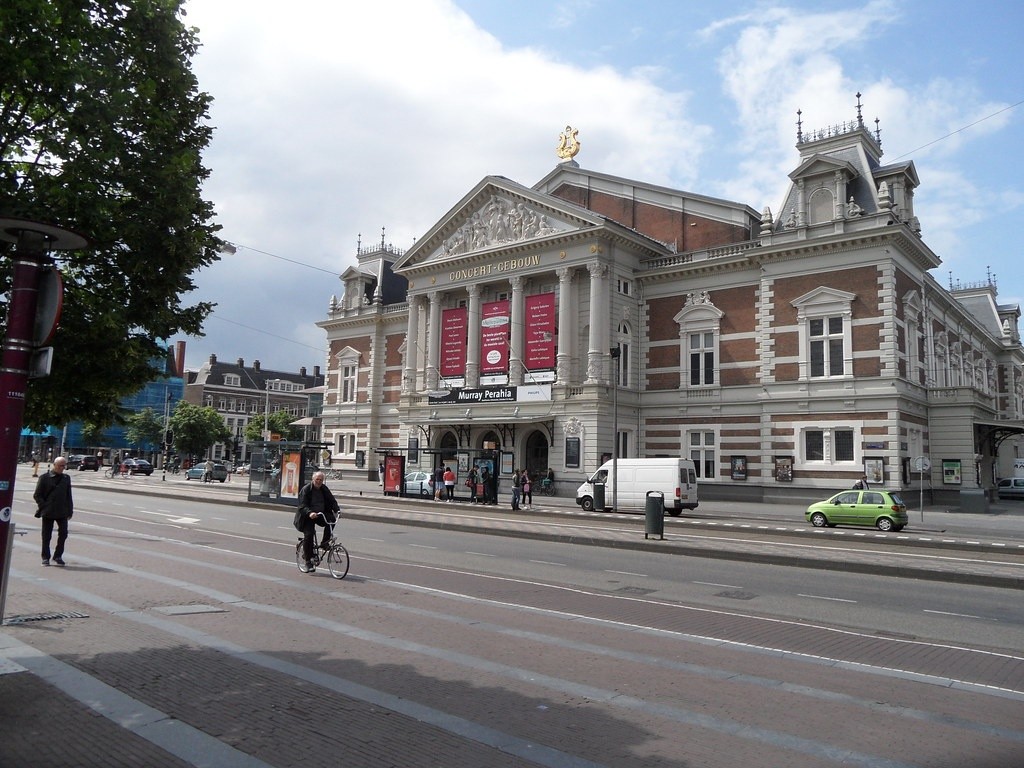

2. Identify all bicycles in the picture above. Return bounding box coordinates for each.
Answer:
[531,478,555,496]
[169,464,181,475]
[199,470,215,483]
[296,512,349,580]
[105,465,134,480]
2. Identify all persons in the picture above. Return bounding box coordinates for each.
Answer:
[547,468,554,486]
[204,458,214,481]
[520,468,533,510]
[468,465,479,504]
[378,463,385,486]
[443,467,455,503]
[511,469,521,510]
[113,453,120,475]
[481,467,492,505]
[293,471,340,572]
[442,194,553,257]
[853,475,869,490]
[434,463,445,501]
[33,457,73,567]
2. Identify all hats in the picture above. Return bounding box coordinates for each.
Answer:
[862,475,867,479]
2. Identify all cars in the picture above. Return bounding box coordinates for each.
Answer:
[185,462,227,483]
[997,478,1024,493]
[404,472,445,499]
[222,460,250,475]
[122,458,154,476]
[804,490,908,532]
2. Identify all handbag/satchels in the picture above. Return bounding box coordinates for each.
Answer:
[465,480,473,487]
[524,484,529,492]
[211,467,215,472]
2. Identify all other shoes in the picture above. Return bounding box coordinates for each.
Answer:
[305,562,315,571]
[42,559,49,566]
[320,543,332,551]
[53,556,65,565]
[513,507,521,510]
[450,499,453,502]
[434,497,440,501]
[446,500,450,502]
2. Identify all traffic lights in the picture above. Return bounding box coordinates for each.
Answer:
[166,430,173,445]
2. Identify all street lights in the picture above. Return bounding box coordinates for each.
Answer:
[264,379,280,441]
[208,395,214,406]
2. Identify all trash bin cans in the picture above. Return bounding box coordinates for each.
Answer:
[644,491,665,534]
[593,481,605,509]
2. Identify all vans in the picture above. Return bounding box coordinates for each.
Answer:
[67,455,98,472]
[576,458,699,516]
